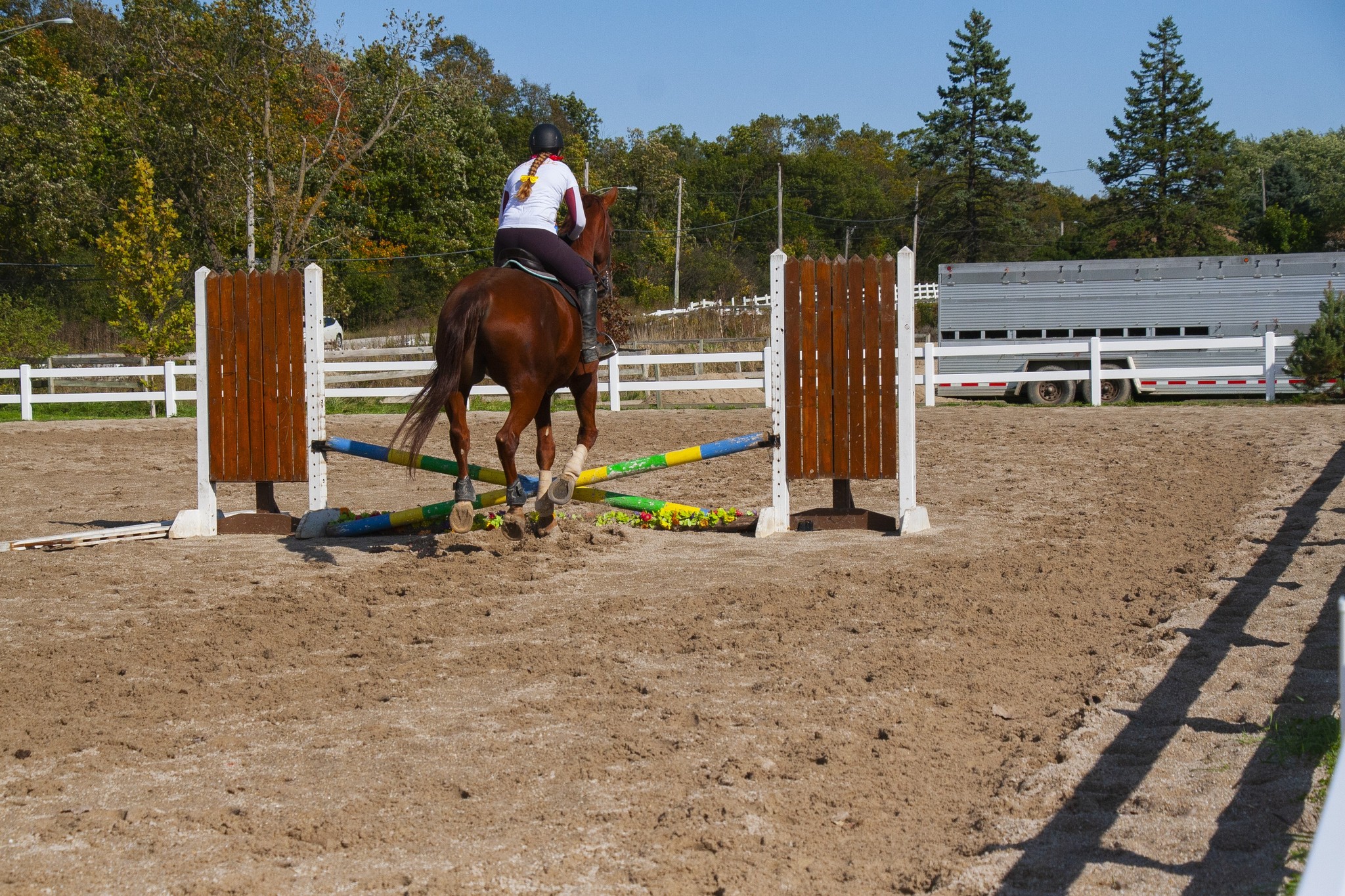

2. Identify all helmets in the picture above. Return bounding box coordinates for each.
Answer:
[528,124,564,151]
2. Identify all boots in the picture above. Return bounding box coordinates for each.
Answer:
[576,279,620,364]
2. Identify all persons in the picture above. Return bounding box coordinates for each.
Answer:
[493,123,619,363]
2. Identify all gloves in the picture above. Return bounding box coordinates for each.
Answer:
[558,229,580,247]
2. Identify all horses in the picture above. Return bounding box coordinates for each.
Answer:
[395,185,619,546]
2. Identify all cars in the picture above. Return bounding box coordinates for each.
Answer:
[290,315,344,351]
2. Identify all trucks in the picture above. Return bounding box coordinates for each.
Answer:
[936,250,1345,407]
